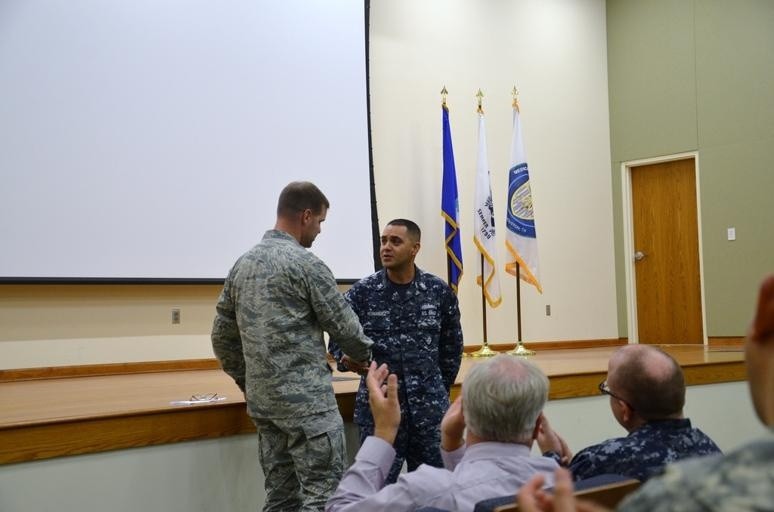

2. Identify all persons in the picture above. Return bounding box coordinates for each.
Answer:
[536,344,725,486]
[515,271,774,512]
[324,352,563,512]
[329,218,464,496]
[210,181,375,512]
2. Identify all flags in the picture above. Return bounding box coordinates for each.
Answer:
[442,101,464,296]
[474,105,503,308]
[504,88,543,295]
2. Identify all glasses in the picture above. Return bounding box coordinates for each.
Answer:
[598,379,624,401]
